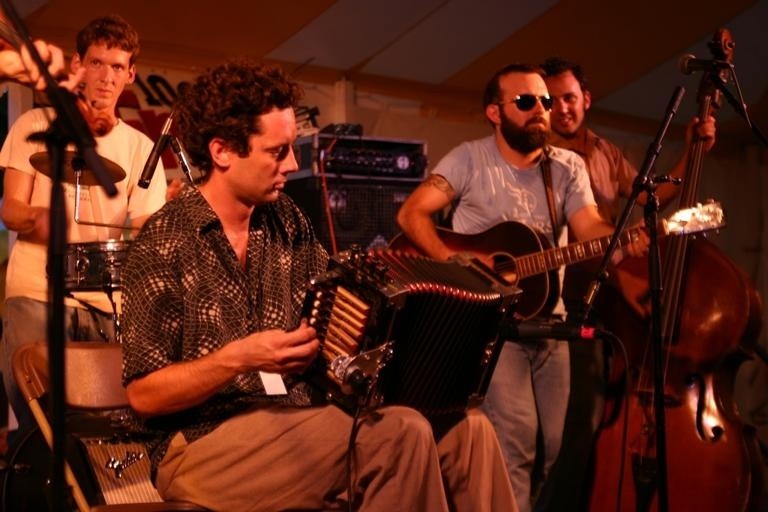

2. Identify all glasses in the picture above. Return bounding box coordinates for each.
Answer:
[498,94,554,111]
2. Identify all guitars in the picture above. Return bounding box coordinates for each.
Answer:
[387,198,726,323]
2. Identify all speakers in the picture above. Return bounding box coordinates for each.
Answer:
[285,178,444,256]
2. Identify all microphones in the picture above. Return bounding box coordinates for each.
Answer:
[505,319,606,342]
[679,53,734,74]
[135,110,175,188]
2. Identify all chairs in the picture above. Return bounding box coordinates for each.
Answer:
[10,339,210,512]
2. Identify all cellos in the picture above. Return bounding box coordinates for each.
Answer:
[563,27,764,510]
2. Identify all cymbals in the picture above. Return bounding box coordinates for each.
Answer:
[29,151,126,185]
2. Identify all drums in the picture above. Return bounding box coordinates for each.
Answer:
[57,239,131,292]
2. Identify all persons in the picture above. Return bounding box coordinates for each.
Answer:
[536,59,717,512]
[396,63,650,512]
[0,13,167,470]
[122,58,521,512]
